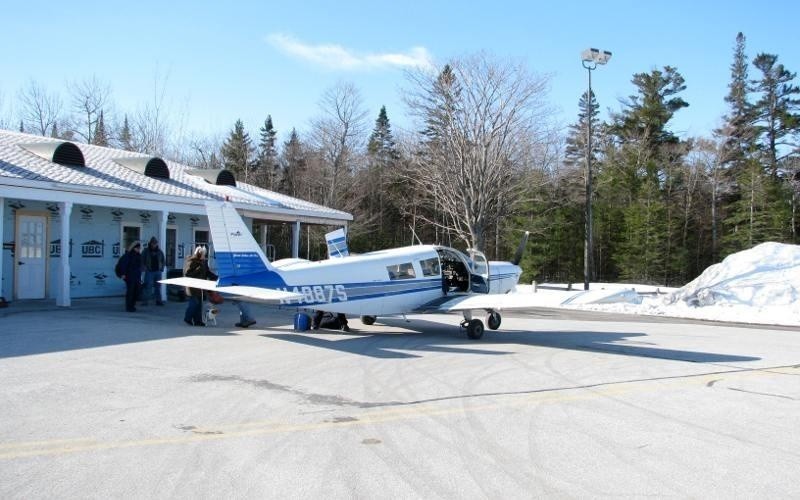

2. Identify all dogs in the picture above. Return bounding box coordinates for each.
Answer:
[204,301,219,327]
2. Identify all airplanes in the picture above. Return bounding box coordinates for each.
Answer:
[159,200,639,340]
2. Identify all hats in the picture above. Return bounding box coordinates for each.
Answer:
[150,236,158,244]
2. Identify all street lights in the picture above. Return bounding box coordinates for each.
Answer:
[582,48,611,289]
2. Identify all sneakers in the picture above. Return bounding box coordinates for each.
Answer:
[184,318,193,326]
[126,301,164,312]
[194,321,205,326]
[235,319,256,328]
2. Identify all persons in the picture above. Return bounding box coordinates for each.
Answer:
[182,246,217,326]
[142,237,166,306]
[115,241,141,312]
